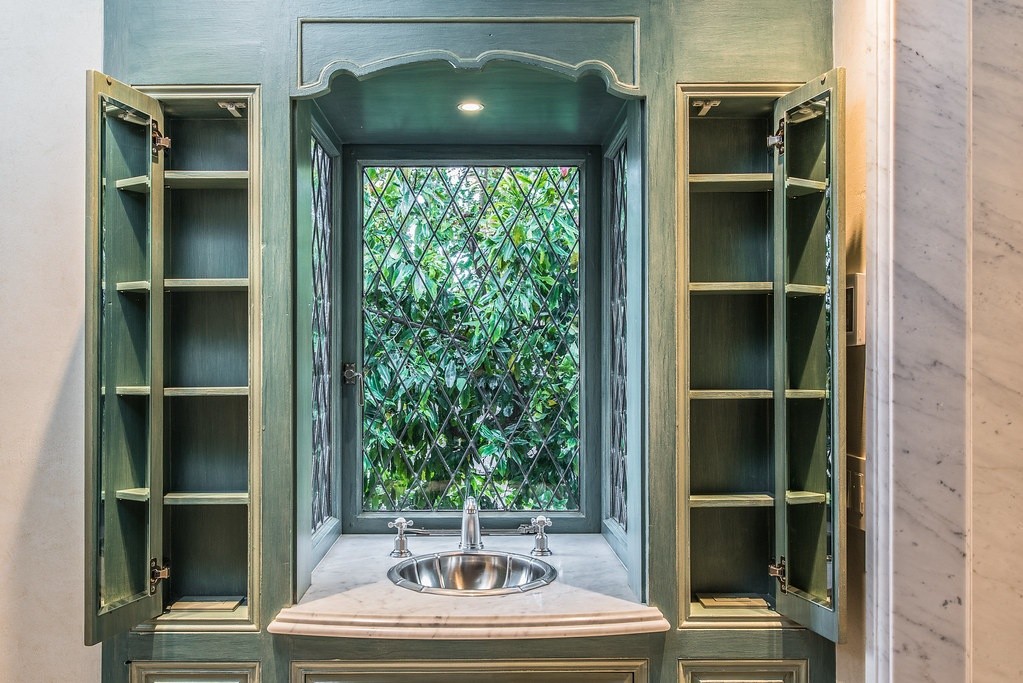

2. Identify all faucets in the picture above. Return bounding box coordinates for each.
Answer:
[458,496,485,550]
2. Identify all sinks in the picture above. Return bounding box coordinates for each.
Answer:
[386,549,558,597]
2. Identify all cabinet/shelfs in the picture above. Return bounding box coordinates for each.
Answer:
[82,69,263,647]
[672,66,850,643]
